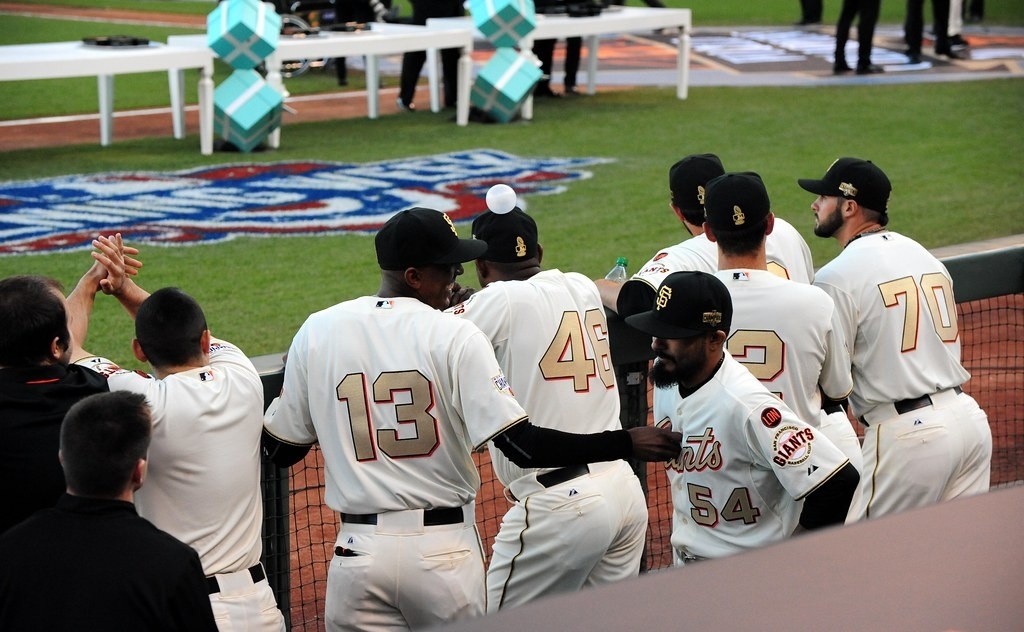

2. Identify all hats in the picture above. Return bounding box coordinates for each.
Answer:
[375,207,488,270]
[626,272,734,338]
[471,207,539,263]
[706,170,771,230]
[798,156,893,213]
[669,153,725,212]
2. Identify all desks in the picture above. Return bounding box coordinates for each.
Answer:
[0,5,693,156]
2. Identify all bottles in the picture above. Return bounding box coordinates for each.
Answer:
[605,257,628,282]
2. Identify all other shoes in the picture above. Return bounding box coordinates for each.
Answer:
[535,89,560,98]
[856,61,884,74]
[564,85,581,94]
[933,40,968,60]
[833,59,853,73]
[396,98,415,114]
[909,54,923,65]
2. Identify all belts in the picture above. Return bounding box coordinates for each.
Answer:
[509,464,589,501]
[340,507,463,526]
[204,562,263,592]
[822,402,843,415]
[857,384,963,428]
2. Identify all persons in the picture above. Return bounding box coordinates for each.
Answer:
[0,275,111,538]
[260,207,681,632]
[592,150,995,525]
[624,266,862,567]
[393,0,467,113]
[445,207,653,613]
[0,390,221,632]
[64,228,290,632]
[264,0,392,88]
[902,0,984,64]
[793,0,824,27]
[531,0,682,99]
[832,0,885,75]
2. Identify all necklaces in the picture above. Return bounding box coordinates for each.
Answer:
[677,353,725,397]
[843,226,885,248]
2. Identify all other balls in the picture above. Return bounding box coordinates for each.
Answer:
[486,183,517,214]
